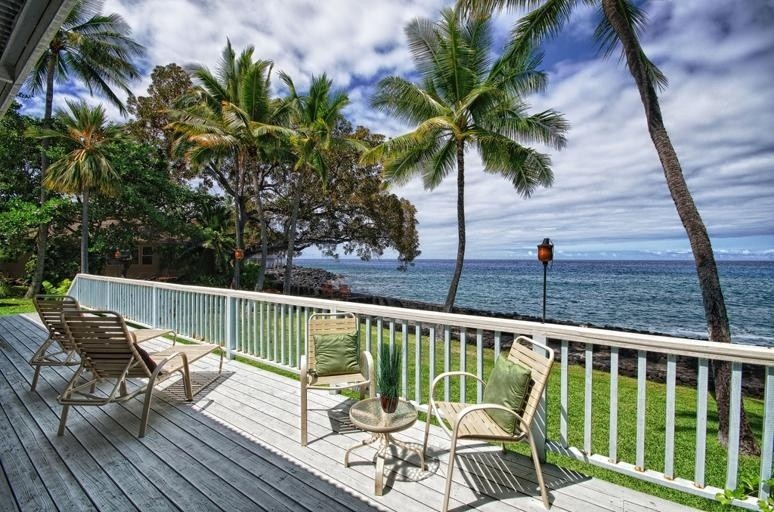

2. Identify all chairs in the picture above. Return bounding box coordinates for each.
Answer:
[424,335,555,512]
[300,312,375,446]
[55,310,223,438]
[28,294,177,393]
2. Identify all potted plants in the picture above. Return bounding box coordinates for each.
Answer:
[371,342,403,414]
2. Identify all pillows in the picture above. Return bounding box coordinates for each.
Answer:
[482,351,532,437]
[313,331,361,377]
